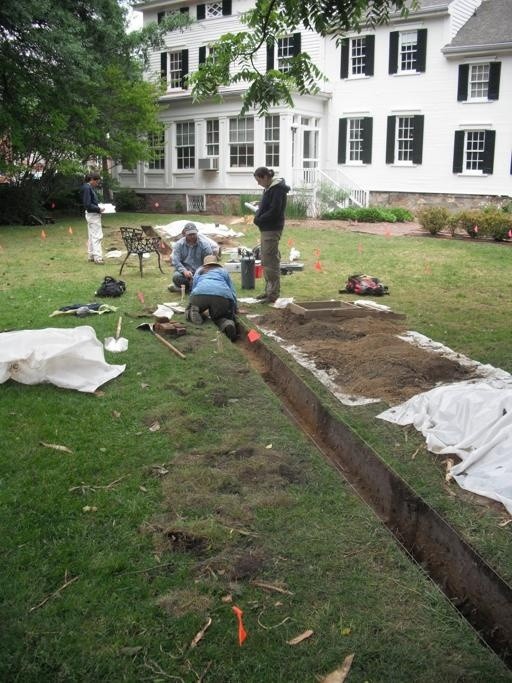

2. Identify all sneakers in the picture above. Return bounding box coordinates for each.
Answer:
[88,258,104,264]
[255,294,276,304]
[167,283,180,291]
[225,324,235,342]
[190,305,203,324]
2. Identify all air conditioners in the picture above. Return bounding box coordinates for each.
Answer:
[198,157,218,171]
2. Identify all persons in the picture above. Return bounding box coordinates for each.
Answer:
[253,166,287,304]
[170,223,238,341]
[81,174,105,264]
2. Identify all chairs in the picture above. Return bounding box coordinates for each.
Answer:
[119,226,164,278]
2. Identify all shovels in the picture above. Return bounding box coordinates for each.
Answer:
[136,323,187,361]
[104,316,129,354]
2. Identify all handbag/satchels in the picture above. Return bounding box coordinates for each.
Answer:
[96,277,126,297]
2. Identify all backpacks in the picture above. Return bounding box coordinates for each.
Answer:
[346,276,386,295]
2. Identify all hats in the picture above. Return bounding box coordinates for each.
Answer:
[204,255,223,268]
[185,223,197,235]
